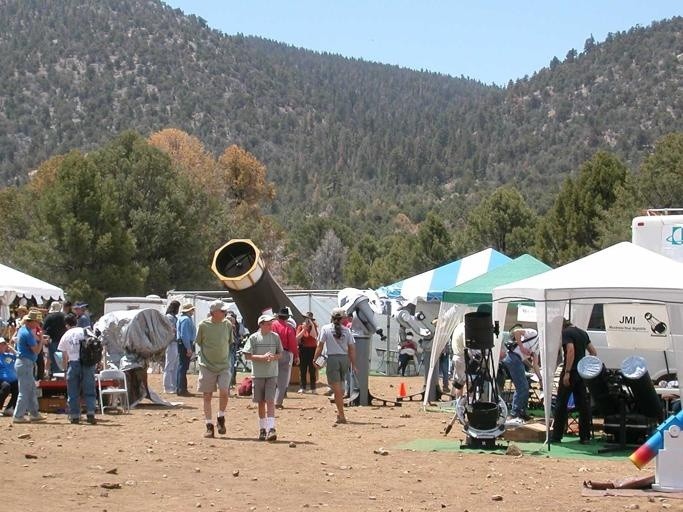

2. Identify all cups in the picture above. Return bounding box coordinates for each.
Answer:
[43,335,48,340]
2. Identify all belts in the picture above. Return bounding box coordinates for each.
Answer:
[441,352,448,355]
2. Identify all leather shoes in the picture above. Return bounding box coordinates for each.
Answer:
[177,388,195,396]
[184,385,187,391]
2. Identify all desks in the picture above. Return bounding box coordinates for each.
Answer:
[376,349,399,375]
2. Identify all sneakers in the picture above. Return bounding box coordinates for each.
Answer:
[164,386,175,393]
[71,418,79,424]
[443,386,450,392]
[217,416,226,434]
[204,423,214,438]
[267,428,277,441]
[545,438,562,445]
[259,428,267,440]
[324,387,334,396]
[87,415,94,422]
[310,389,317,394]
[513,409,532,421]
[297,388,305,394]
[335,415,346,423]
[579,440,589,445]
[12,417,31,423]
[173,384,177,389]
[29,412,47,421]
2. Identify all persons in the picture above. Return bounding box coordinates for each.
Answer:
[449,322,465,401]
[162,301,180,394]
[285,318,300,399]
[42,301,66,371]
[195,299,233,439]
[549,316,597,445]
[430,318,453,393]
[225,310,241,388]
[323,313,347,396]
[272,308,300,409]
[176,304,196,397]
[496,324,522,400]
[9,305,28,350]
[343,313,352,398]
[296,312,318,394]
[12,310,48,424]
[242,315,284,441]
[57,313,104,424]
[0,336,18,416]
[63,301,77,318]
[313,308,359,424]
[503,328,542,422]
[398,332,418,377]
[72,304,91,328]
[36,311,45,380]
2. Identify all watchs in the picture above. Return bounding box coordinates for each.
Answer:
[564,370,571,373]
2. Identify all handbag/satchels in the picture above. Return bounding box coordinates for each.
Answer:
[505,332,538,352]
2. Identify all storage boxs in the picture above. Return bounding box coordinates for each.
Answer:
[37,397,66,413]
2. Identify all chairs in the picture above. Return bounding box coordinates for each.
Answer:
[398,346,419,375]
[98,370,129,416]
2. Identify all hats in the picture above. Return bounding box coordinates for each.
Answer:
[181,303,197,313]
[72,301,88,308]
[210,300,231,312]
[31,310,42,320]
[0,337,5,343]
[278,308,289,318]
[25,310,37,321]
[64,302,71,306]
[302,312,315,321]
[258,315,275,325]
[330,307,345,318]
[15,305,28,313]
[551,316,568,324]
[49,302,60,314]
[408,332,413,335]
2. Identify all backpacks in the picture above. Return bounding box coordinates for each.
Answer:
[80,327,103,366]
